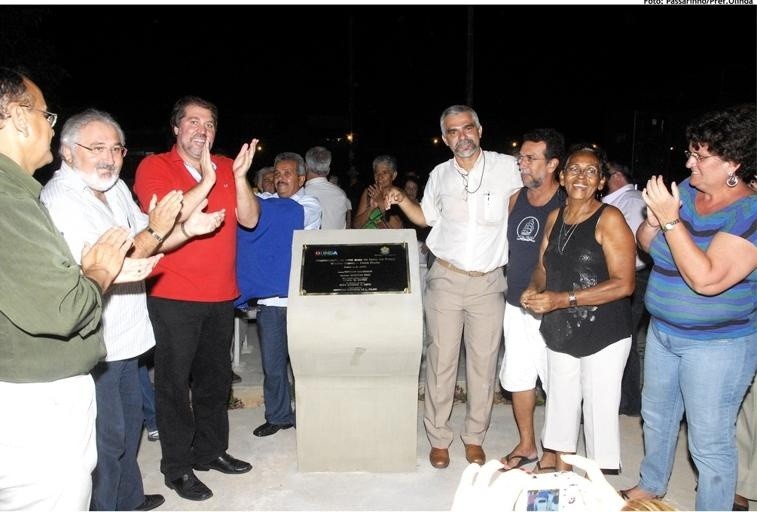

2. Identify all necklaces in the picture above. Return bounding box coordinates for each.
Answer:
[557,198,596,256]
[454,150,486,194]
[563,220,577,236]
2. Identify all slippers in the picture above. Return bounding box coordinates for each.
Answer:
[498,453,557,474]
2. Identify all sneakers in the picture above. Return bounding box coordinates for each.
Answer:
[147,428,161,443]
[231,370,241,383]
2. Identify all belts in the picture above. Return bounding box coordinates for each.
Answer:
[434,255,501,279]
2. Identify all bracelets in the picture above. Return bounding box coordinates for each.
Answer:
[146,226,164,245]
[181,222,192,241]
[568,288,579,308]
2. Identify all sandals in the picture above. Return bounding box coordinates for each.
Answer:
[618,488,664,502]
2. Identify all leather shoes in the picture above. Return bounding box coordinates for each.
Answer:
[429,446,451,470]
[192,451,253,475]
[252,420,294,438]
[465,443,487,466]
[129,494,165,511]
[165,469,213,501]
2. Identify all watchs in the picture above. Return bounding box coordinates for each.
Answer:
[661,217,681,233]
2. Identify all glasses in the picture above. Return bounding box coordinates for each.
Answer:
[72,142,128,158]
[684,150,718,164]
[18,103,59,129]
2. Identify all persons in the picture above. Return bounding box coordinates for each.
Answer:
[614,104,756,510]
[619,497,677,510]
[40,106,227,511]
[139,145,352,444]
[518,145,638,476]
[380,103,523,470]
[496,127,566,475]
[1,62,166,510]
[353,154,466,404]
[599,147,647,419]
[732,373,756,511]
[132,94,262,501]
